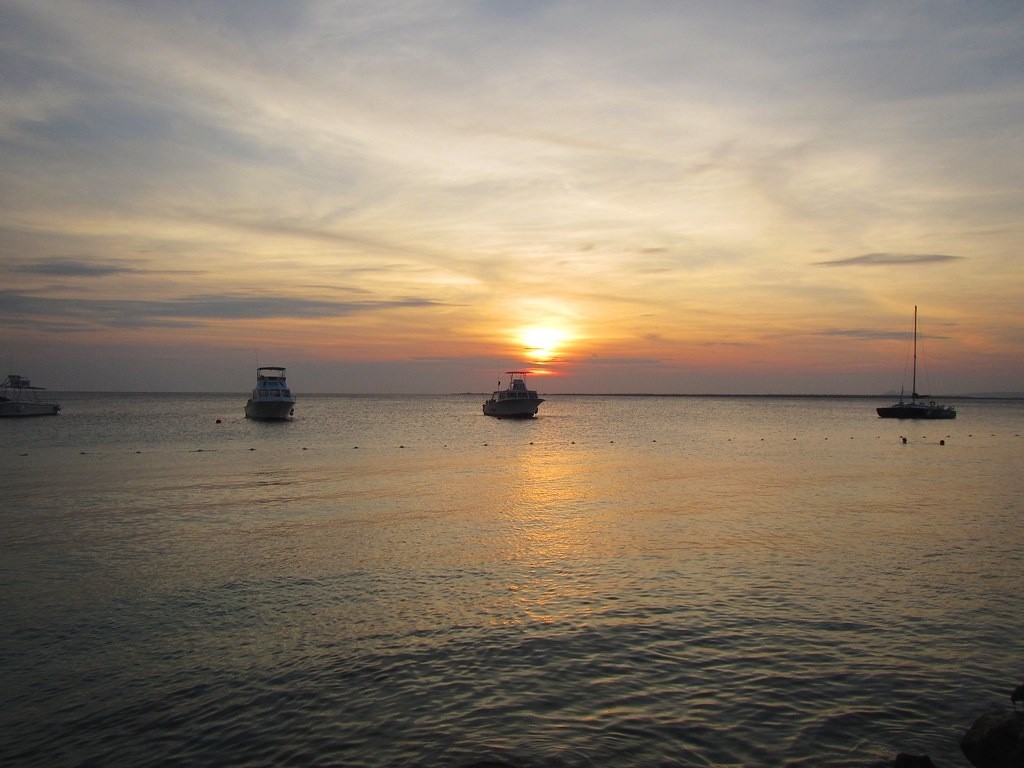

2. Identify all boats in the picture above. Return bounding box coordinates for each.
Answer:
[0,374,62,418]
[482,372,546,419]
[245,366,297,420]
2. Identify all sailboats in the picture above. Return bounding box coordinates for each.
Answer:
[876,306,957,419]
